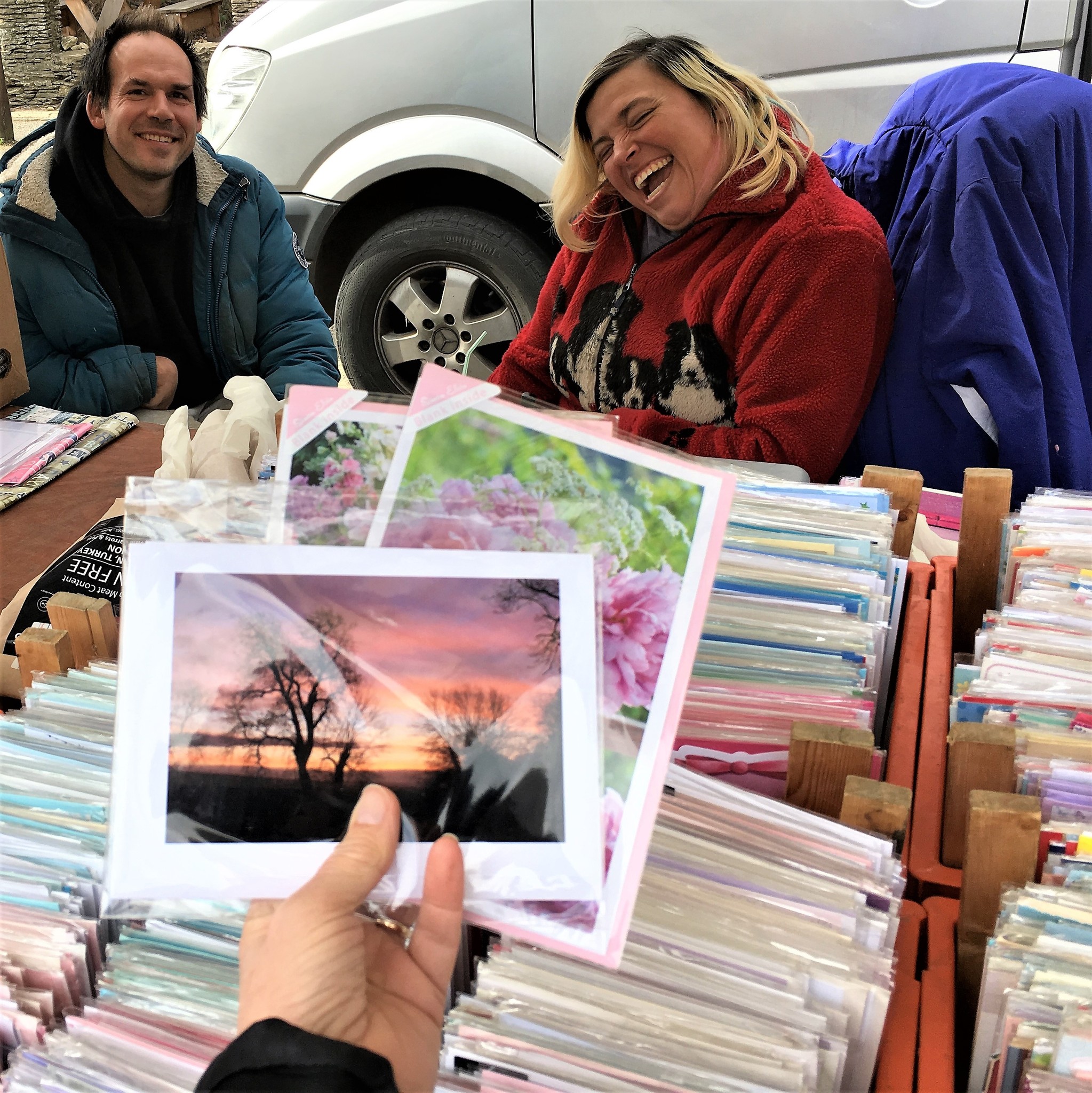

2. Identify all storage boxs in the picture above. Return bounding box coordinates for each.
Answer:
[861,554,964,1093]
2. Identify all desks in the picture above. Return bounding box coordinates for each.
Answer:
[1,424,195,616]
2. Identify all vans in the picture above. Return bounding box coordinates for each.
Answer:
[204,1,1092,395]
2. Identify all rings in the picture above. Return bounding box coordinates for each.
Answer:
[376,918,408,933]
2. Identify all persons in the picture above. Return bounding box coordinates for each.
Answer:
[0,2,341,423]
[192,784,464,1093]
[485,34,897,483]
